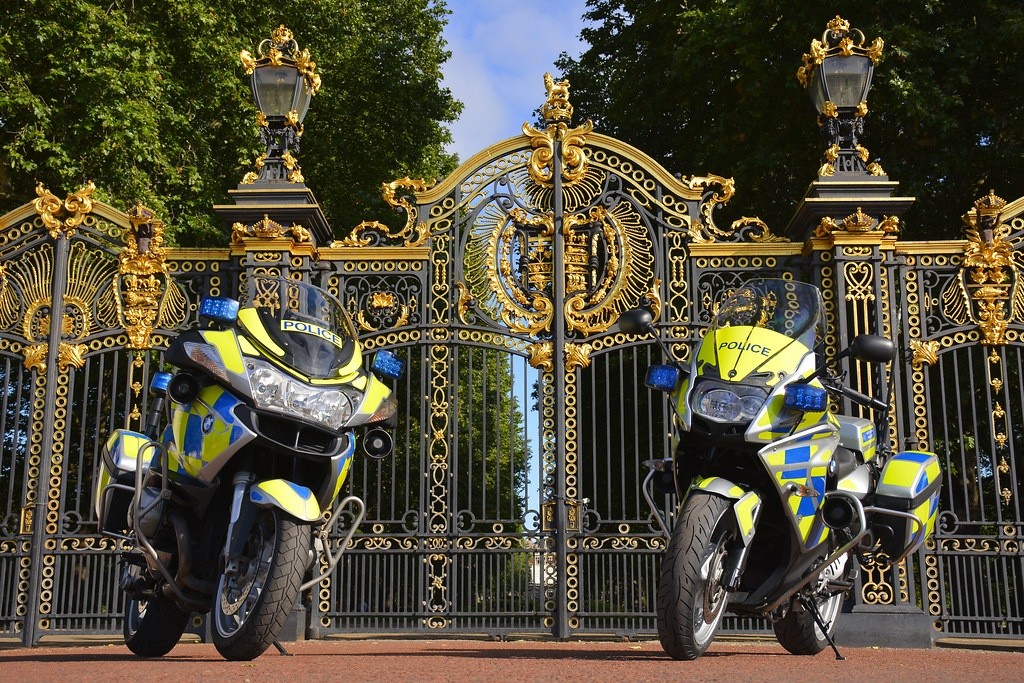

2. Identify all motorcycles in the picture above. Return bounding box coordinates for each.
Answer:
[617,277,944,661]
[91,276,407,661]
